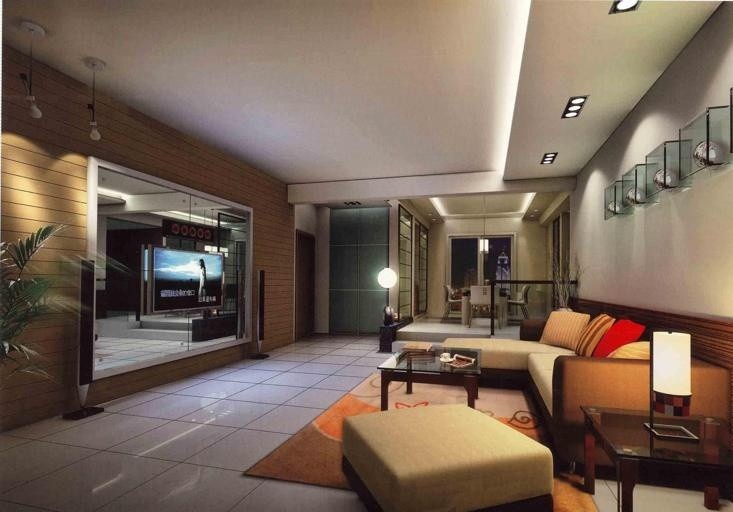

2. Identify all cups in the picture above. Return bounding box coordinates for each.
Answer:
[440,352,450,360]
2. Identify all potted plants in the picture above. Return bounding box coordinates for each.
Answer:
[550,255,581,312]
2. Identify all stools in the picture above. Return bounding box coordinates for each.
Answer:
[338,402,556,510]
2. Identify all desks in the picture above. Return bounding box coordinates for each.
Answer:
[578,403,733,510]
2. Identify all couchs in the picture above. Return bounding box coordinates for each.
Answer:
[441,318,731,467]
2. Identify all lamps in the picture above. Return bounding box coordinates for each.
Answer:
[642,328,700,442]
[20,20,49,121]
[82,56,107,142]
[376,267,398,325]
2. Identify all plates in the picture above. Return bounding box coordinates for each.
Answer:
[606,201,627,219]
[439,358,453,362]
[653,168,679,193]
[625,186,648,208]
[693,139,723,170]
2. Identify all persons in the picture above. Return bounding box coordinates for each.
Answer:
[198,259,206,302]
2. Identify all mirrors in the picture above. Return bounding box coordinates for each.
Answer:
[88,155,253,380]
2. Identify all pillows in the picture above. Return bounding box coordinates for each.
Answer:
[590,318,647,356]
[573,312,616,356]
[605,340,651,359]
[538,310,591,352]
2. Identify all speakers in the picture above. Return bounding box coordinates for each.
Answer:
[63,259,105,420]
[248,269,269,359]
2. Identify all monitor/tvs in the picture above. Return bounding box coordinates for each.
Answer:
[147,244,225,315]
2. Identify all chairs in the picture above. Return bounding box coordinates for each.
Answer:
[440,284,530,329]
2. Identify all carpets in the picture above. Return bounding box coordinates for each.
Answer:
[242,368,598,511]
